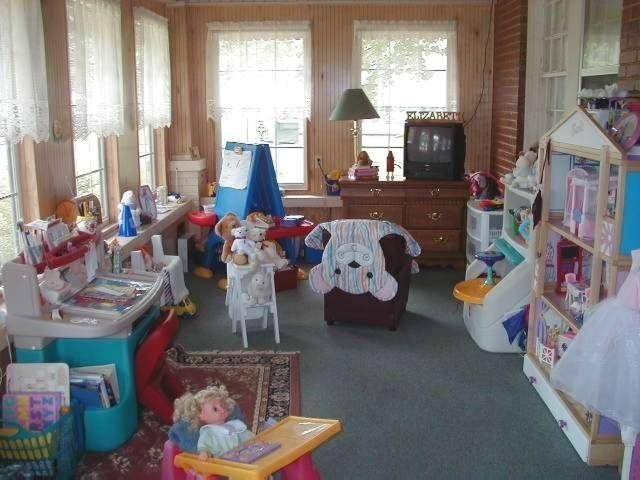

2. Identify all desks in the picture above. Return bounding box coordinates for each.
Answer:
[1,246,165,452]
[102,196,194,262]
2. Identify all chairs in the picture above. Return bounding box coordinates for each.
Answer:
[134,307,187,427]
[160,416,343,479]
[317,219,413,332]
[223,220,281,347]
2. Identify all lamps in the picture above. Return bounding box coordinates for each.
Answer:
[329,88,379,167]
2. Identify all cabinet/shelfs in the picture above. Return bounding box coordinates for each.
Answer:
[464,199,504,273]
[521,105,640,468]
[338,174,468,273]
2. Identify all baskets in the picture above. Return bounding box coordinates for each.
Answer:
[0,399,87,479]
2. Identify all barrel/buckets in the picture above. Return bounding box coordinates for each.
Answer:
[324,179,340,196]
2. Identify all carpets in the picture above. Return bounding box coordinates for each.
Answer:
[70,350,302,480]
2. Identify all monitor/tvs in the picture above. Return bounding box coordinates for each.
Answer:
[404,123,466,181]
